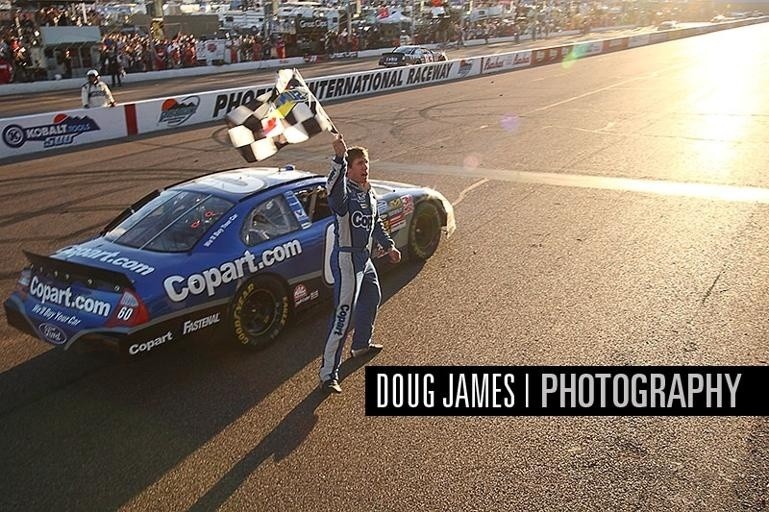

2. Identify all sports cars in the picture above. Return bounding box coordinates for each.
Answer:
[657,20,682,30]
[378,46,448,67]
[4,164,455,365]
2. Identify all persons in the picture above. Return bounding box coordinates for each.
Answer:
[1,0,449,86]
[449,0,768,47]
[76,69,117,107]
[317,134,402,395]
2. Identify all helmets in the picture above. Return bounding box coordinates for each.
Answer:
[86,70,101,85]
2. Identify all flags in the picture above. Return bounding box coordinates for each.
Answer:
[224,67,342,164]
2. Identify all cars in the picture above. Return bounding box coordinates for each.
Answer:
[710,13,746,23]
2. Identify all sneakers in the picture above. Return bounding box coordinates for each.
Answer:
[321,379,345,394]
[349,341,383,357]
